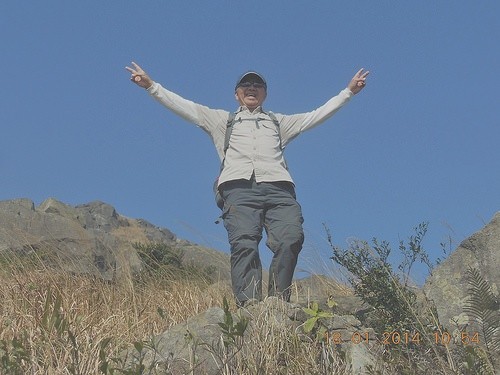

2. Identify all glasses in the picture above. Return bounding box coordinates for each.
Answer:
[237,80,266,87]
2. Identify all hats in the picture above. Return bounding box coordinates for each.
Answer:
[235,71,268,91]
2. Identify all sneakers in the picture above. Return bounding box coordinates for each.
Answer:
[243,296,280,308]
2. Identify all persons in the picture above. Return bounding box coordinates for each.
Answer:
[125,62,369,308]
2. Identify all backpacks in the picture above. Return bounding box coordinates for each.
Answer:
[213,111,282,210]
